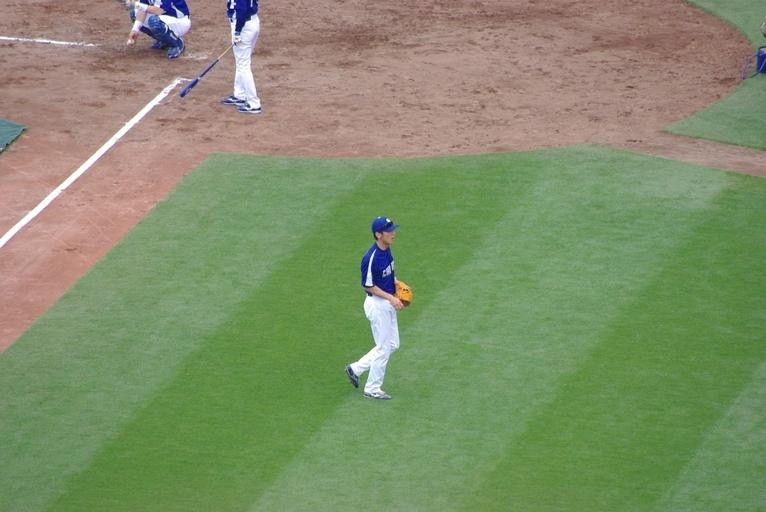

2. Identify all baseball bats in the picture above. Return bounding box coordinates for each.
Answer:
[180,43,234,97]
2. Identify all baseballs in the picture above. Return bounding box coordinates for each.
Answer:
[128,39,134,46]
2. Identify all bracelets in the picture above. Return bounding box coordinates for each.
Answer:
[135,2,148,12]
[132,20,143,33]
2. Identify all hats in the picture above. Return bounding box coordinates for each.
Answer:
[372,217,400,232]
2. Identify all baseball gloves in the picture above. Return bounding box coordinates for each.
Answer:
[394,281,412,306]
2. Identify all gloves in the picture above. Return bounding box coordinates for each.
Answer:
[233,31,242,46]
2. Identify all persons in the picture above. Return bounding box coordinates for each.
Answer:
[126,0,191,60]
[222,0,262,114]
[345,216,404,400]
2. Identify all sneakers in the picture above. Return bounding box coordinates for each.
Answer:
[150,38,185,58]
[222,95,262,114]
[363,391,391,400]
[345,364,358,389]
